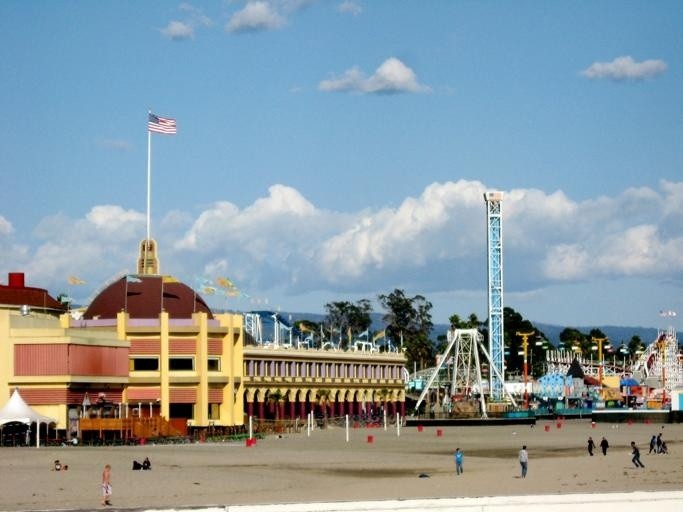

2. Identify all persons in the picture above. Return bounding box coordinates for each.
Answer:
[630,441,644,468]
[142,456,150,469]
[101,464,113,507]
[518,445,528,477]
[587,436,596,456]
[648,432,669,454]
[51,459,63,470]
[61,436,67,446]
[453,447,464,475]
[71,435,78,445]
[599,437,608,455]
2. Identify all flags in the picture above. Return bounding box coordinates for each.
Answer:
[148,112,177,136]
